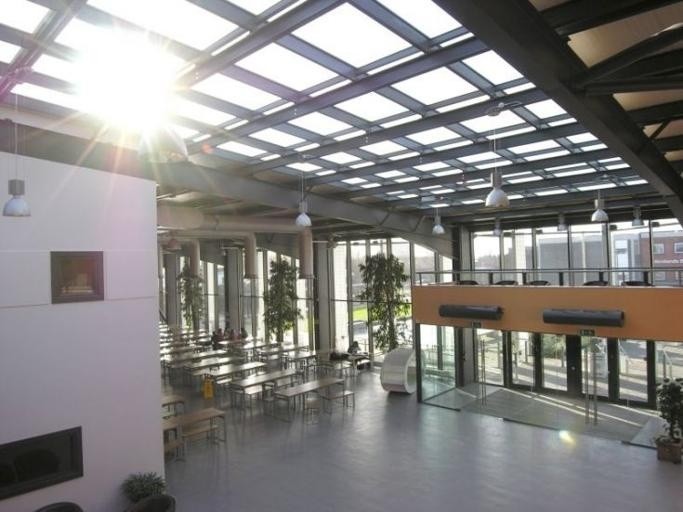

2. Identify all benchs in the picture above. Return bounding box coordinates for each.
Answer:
[164,439,183,467]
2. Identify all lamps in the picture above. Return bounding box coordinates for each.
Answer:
[3,76,31,218]
[295,161,311,227]
[430,103,610,235]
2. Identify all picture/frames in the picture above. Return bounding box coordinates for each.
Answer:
[49,249,105,305]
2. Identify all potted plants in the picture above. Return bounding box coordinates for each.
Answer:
[653,377,683,463]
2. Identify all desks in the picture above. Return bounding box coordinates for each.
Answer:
[159,323,369,443]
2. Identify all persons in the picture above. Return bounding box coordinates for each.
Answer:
[348,341,361,354]
[211,327,248,350]
